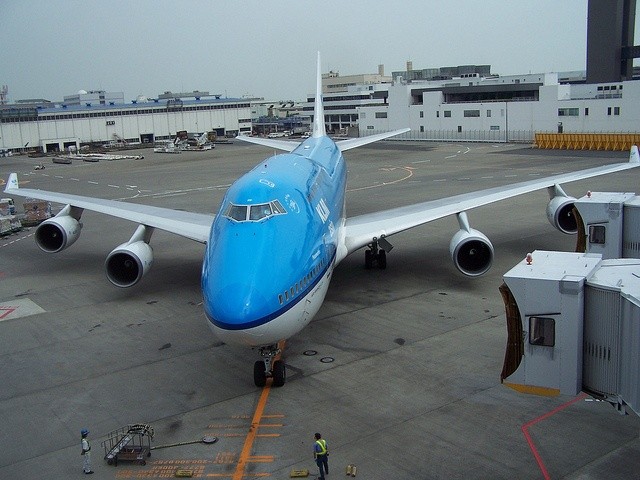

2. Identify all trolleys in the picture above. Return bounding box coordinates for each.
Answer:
[99,423,156,465]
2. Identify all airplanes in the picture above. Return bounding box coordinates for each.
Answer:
[4,51,640,386]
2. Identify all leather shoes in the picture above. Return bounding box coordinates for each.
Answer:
[85,471,94,474]
[318,477,325,480]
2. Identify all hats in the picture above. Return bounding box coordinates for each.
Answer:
[81,429,89,435]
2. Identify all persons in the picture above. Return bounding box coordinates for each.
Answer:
[312,433,330,479]
[80,429,94,475]
